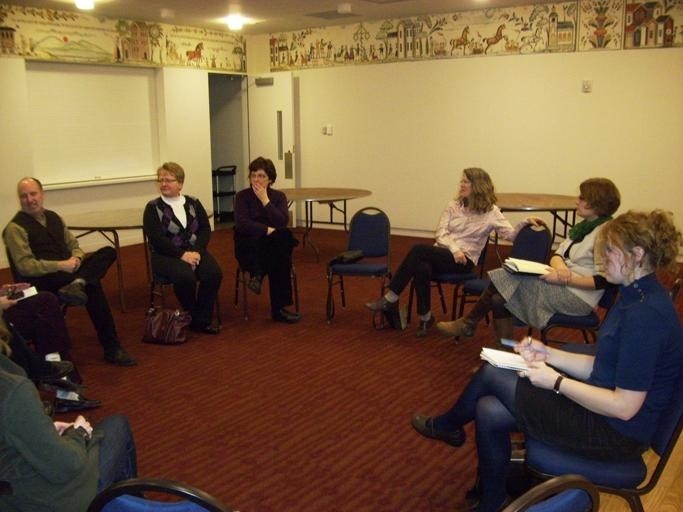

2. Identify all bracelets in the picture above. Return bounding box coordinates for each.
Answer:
[553,373,567,398]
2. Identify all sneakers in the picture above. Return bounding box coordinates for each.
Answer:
[107,346,136,366]
[58,283,89,305]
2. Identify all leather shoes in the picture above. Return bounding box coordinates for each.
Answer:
[415,314,434,337]
[271,307,303,323]
[363,297,399,313]
[37,360,101,417]
[248,269,263,295]
[411,412,465,447]
[201,323,223,334]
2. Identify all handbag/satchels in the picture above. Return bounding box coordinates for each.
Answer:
[384,289,408,330]
[328,250,364,265]
[142,305,191,344]
[467,439,540,505]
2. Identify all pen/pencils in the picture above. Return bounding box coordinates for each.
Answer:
[500,338,552,355]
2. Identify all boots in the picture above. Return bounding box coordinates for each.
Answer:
[437,289,493,337]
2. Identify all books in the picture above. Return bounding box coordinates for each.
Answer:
[479,345,547,373]
[502,255,554,276]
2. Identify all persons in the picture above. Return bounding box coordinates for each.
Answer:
[435,178,620,338]
[364,165,546,338]
[232,155,302,322]
[0,311,140,511]
[1,176,137,367]
[142,160,224,334]
[409,209,683,512]
[1,278,103,412]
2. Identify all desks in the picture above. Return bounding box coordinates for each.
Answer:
[478,191,581,260]
[264,187,372,249]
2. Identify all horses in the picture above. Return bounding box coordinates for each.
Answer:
[482,23,506,52]
[184,42,205,66]
[446,26,470,56]
